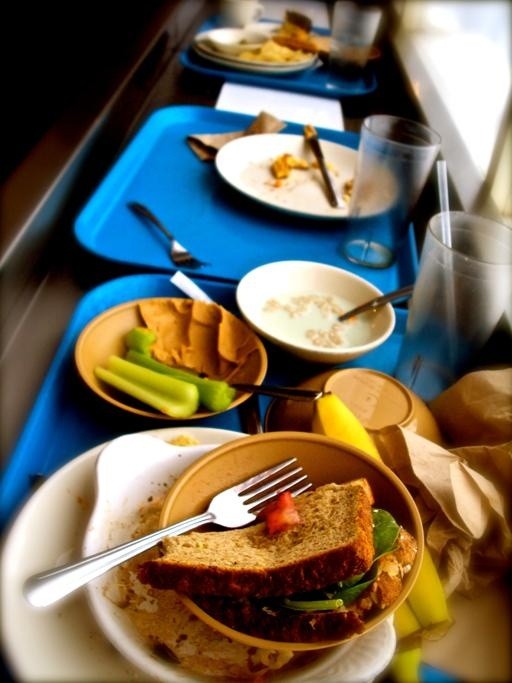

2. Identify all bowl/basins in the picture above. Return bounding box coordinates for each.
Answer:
[82,431,358,682]
[206,28,268,54]
[158,431,424,653]
[72,296,268,422]
[236,259,397,363]
[264,366,446,448]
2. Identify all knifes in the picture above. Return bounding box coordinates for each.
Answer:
[304,123,342,207]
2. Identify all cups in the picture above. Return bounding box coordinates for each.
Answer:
[218,0,265,28]
[325,0,383,90]
[338,114,442,270]
[394,210,512,409]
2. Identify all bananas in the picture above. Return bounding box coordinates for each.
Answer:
[311,392,454,682]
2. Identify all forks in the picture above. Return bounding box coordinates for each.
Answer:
[24,455,314,609]
[126,201,209,270]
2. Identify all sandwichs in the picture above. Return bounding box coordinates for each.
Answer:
[139,475,419,645]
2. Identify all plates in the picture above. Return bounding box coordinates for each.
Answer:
[0,424,394,683]
[195,50,309,77]
[313,37,379,60]
[214,132,401,220]
[193,28,317,68]
